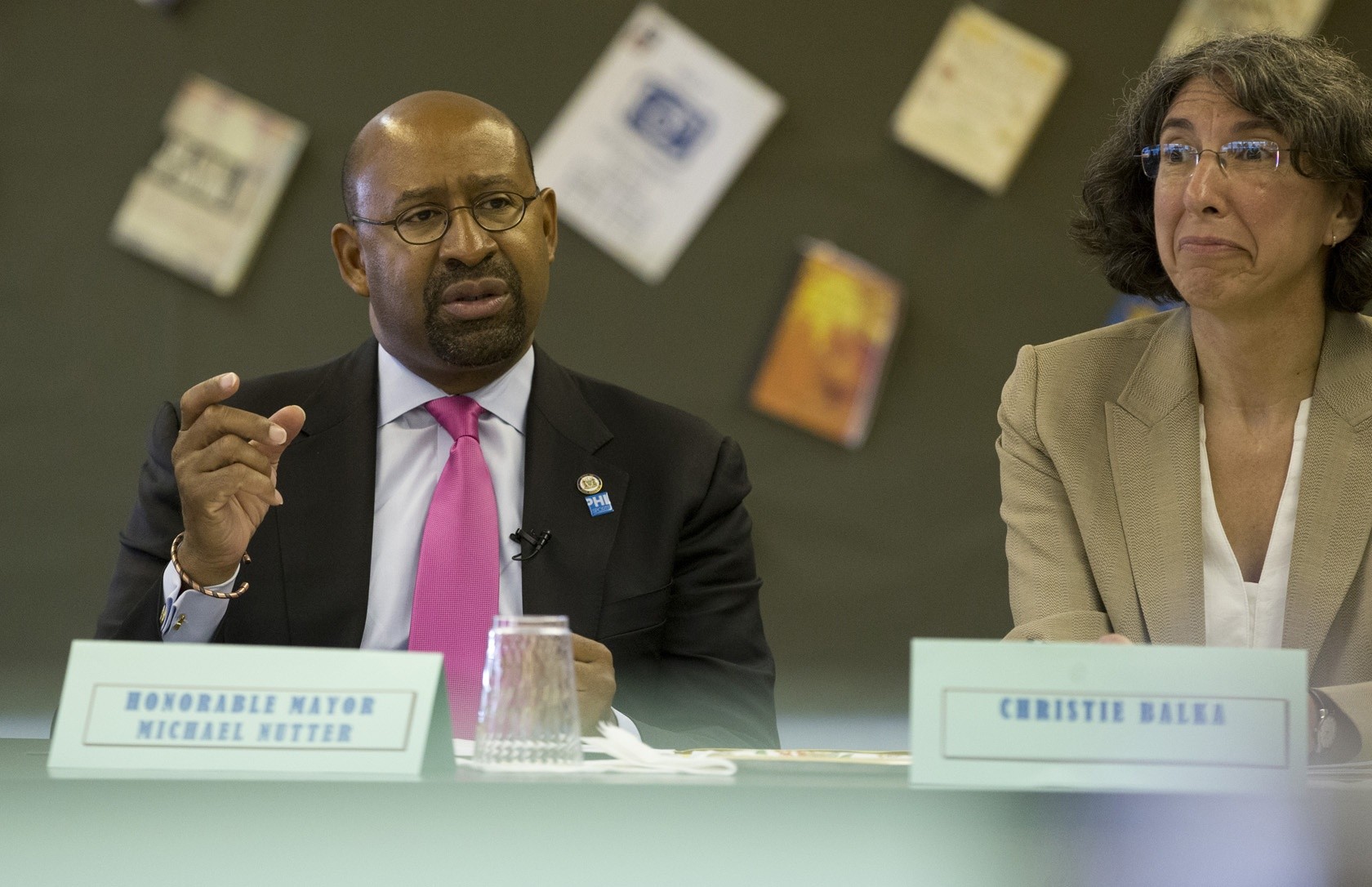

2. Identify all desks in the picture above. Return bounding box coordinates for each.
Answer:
[0,740,1371,886]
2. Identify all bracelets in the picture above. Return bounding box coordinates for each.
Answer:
[170,531,250,599]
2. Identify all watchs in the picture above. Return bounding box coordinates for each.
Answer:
[1307,689,1338,763]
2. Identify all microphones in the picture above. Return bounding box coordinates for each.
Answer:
[536,528,552,551]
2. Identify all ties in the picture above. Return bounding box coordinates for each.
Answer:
[421,395,500,740]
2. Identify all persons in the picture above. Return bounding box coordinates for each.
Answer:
[994,35,1372,765]
[101,89,783,760]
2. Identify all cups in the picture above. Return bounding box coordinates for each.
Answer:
[471,614,581,770]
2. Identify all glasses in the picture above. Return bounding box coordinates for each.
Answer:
[353,190,543,247]
[1133,136,1313,179]
